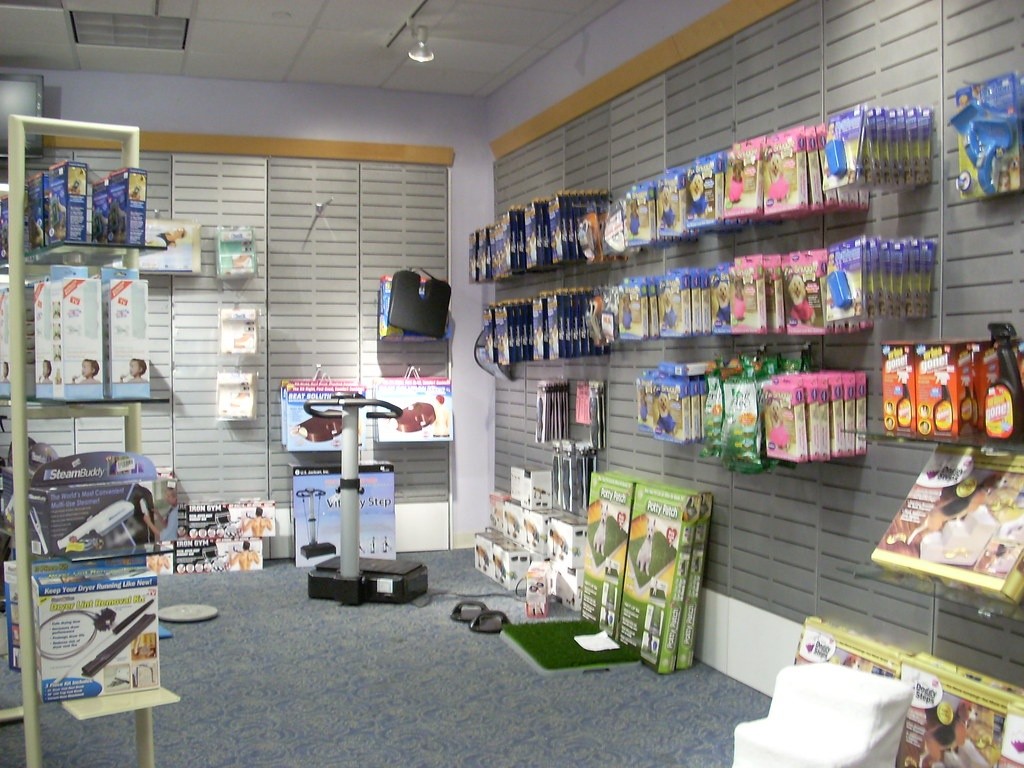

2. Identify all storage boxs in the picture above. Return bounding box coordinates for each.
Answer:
[761,338,1024,768]
[0,265,150,401]
[474,464,713,675]
[0,436,276,703]
[0,160,148,257]
[287,461,396,567]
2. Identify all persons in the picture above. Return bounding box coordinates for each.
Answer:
[143,486,272,573]
[39,360,53,385]
[2,361,11,384]
[72,359,102,385]
[120,359,149,383]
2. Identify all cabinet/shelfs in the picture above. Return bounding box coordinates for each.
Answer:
[0,114,156,768]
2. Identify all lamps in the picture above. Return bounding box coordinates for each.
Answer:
[406,16,435,62]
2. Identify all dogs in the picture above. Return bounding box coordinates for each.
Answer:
[593,504,609,556]
[636,519,656,576]
